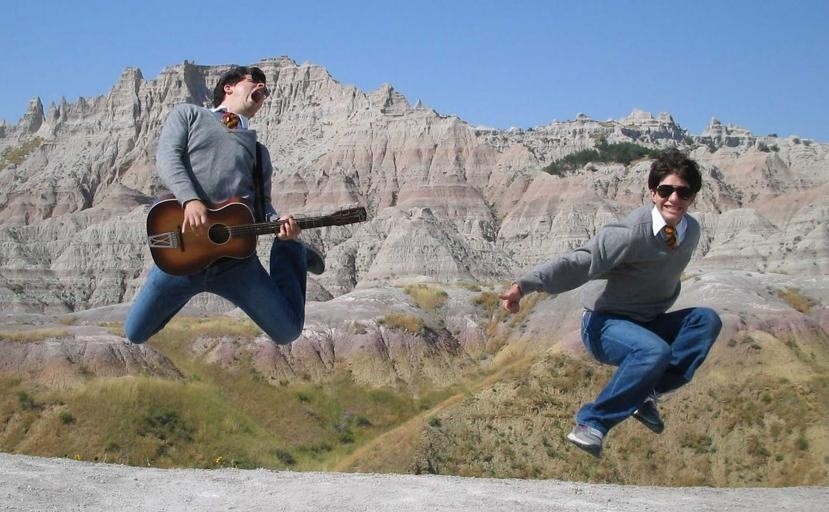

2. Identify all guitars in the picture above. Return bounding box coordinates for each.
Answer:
[146,193,367,276]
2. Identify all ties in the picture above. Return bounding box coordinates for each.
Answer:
[221,112,239,129]
[664,227,678,247]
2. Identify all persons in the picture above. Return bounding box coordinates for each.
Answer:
[494,147,722,459]
[120,65,325,346]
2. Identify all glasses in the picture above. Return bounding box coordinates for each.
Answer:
[236,73,270,101]
[654,185,696,198]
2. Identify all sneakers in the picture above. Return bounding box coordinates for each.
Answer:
[633,399,664,434]
[567,426,605,460]
[272,235,324,274]
